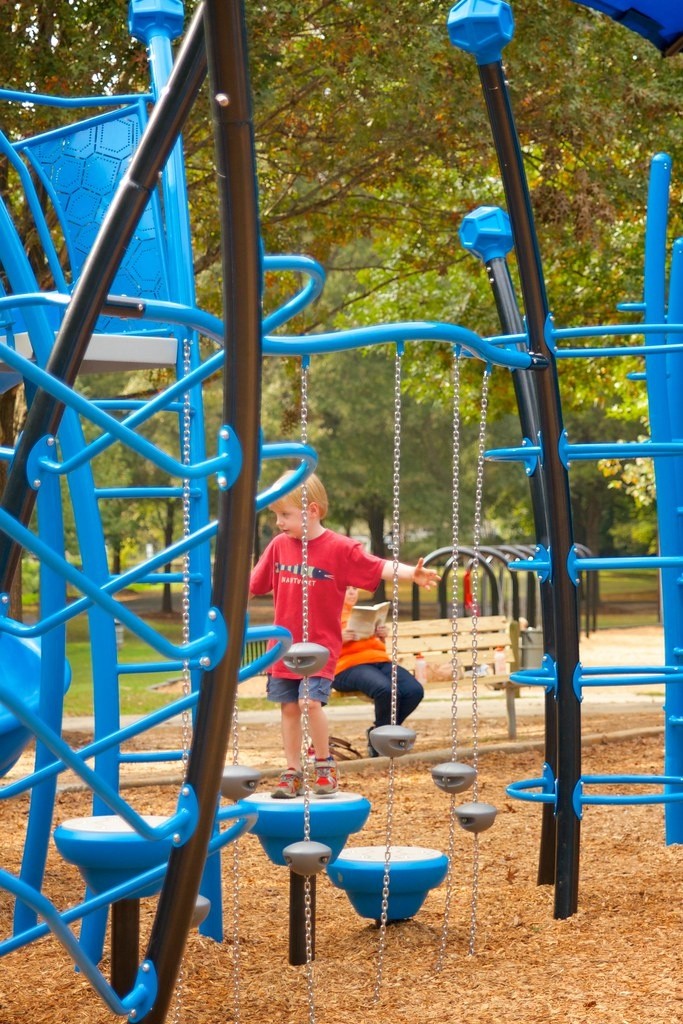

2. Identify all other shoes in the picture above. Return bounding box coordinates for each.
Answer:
[367,726,379,757]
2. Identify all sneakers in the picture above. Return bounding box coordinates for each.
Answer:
[315,758,340,794]
[271,766,305,798]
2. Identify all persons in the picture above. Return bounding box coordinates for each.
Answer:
[329,586,425,759]
[248,469,443,799]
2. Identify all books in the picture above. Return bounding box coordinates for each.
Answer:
[346,601,390,640]
